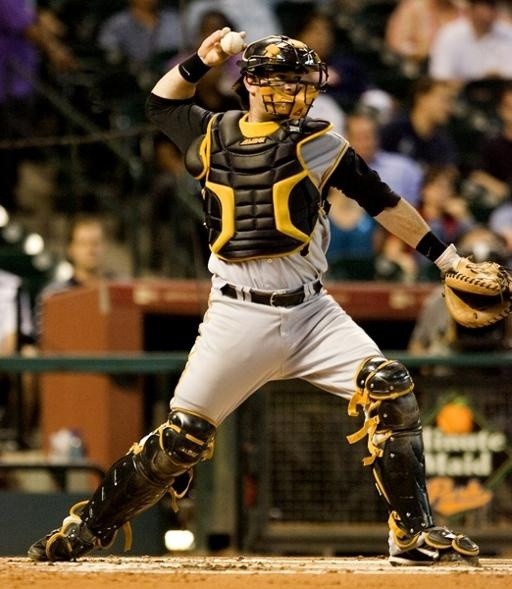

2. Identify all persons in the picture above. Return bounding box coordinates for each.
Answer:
[27,28,511,564]
[1,0,512,451]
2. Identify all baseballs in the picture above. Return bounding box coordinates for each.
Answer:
[222,32,244,57]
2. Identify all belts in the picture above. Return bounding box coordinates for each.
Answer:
[220,281,322,306]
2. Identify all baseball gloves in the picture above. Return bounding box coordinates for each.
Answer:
[446,258,512,328]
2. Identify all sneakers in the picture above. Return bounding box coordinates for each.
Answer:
[28,527,60,561]
[388,527,479,566]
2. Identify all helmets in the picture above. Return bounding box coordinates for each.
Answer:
[236,34,329,142]
